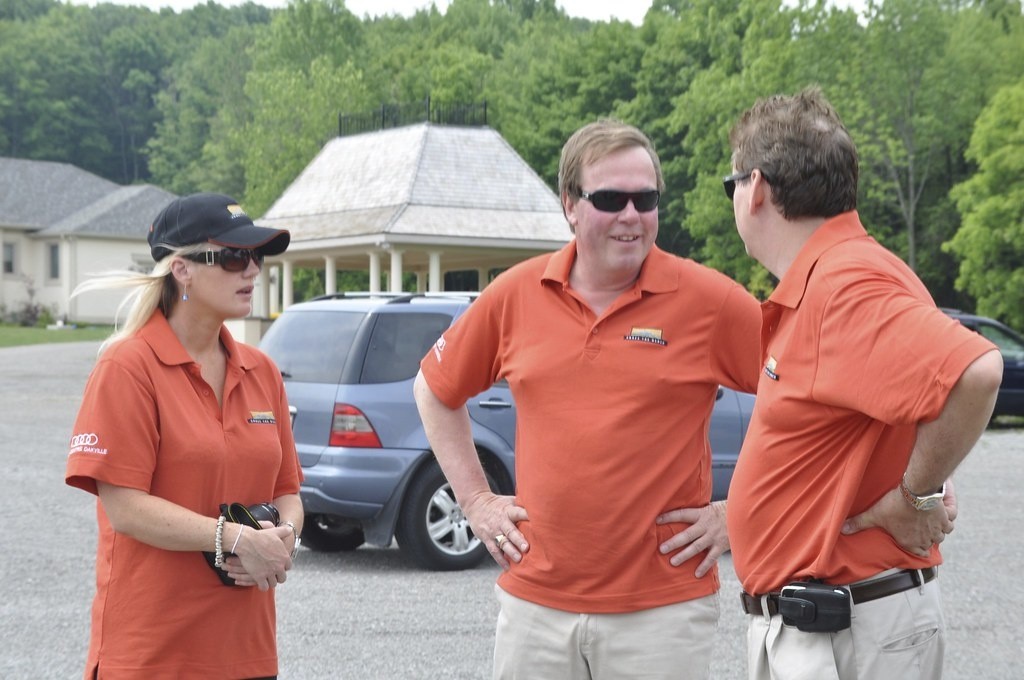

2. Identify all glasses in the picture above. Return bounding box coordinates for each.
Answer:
[182,245,266,274]
[567,185,662,214]
[723,166,779,201]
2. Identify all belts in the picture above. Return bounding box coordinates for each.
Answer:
[739,565,936,629]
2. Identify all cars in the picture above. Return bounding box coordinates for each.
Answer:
[937,306,1024,430]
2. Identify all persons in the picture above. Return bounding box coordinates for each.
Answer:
[724,81,1004,680]
[411,121,761,680]
[65,190,303,678]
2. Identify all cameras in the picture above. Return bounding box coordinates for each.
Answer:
[223,500,280,531]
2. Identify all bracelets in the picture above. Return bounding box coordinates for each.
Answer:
[215,515,225,567]
[232,522,243,552]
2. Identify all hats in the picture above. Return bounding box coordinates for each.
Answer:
[147,192,290,266]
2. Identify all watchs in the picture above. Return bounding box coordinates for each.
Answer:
[280,516,301,559]
[900,472,946,511]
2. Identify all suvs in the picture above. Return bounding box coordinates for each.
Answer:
[249,289,758,572]
